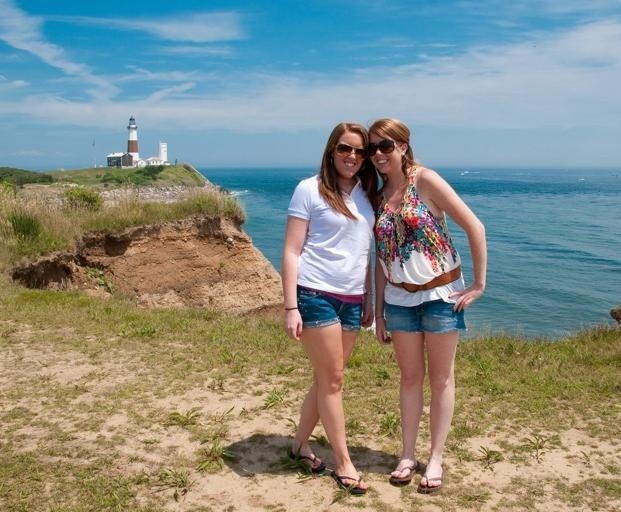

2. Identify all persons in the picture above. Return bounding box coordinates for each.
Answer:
[281,120,380,496]
[364,113,487,496]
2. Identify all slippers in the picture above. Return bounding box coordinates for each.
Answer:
[331,471,366,494]
[390,468,417,482]
[417,469,444,493]
[287,446,325,472]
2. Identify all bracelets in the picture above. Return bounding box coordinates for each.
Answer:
[365,289,374,297]
[283,304,300,312]
[376,314,385,320]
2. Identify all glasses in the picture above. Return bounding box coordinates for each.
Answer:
[367,140,402,157]
[335,144,363,159]
